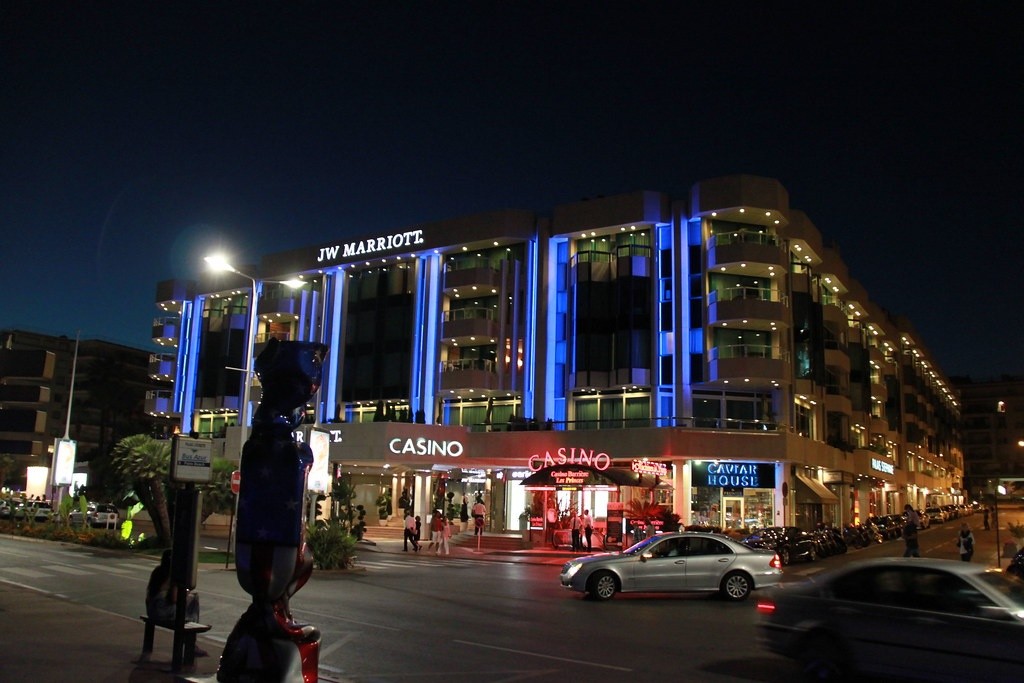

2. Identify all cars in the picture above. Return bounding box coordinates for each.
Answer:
[814,500,985,556]
[752,558,1024,683]
[0,499,18,518]
[1007,546,1024,575]
[559,530,783,602]
[742,526,819,565]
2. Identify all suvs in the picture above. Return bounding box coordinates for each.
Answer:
[13,500,52,523]
[68,501,120,529]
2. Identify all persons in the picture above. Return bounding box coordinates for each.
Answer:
[957,522,976,562]
[402,510,423,552]
[145,550,207,655]
[570,511,582,552]
[473,500,486,535]
[428,511,452,555]
[582,509,594,552]
[983,505,996,531]
[901,503,920,558]
[30,494,46,501]
[656,539,678,556]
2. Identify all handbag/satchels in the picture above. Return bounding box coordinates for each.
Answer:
[414,530,418,535]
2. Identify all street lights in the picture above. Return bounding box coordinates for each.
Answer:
[201,251,307,551]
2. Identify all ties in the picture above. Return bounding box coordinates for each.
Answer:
[573,519,575,530]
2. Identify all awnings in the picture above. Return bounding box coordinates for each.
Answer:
[795,475,838,505]
[519,464,614,485]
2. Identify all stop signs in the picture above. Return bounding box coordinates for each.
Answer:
[230,470,241,495]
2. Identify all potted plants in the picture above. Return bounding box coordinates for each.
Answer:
[375,490,391,526]
